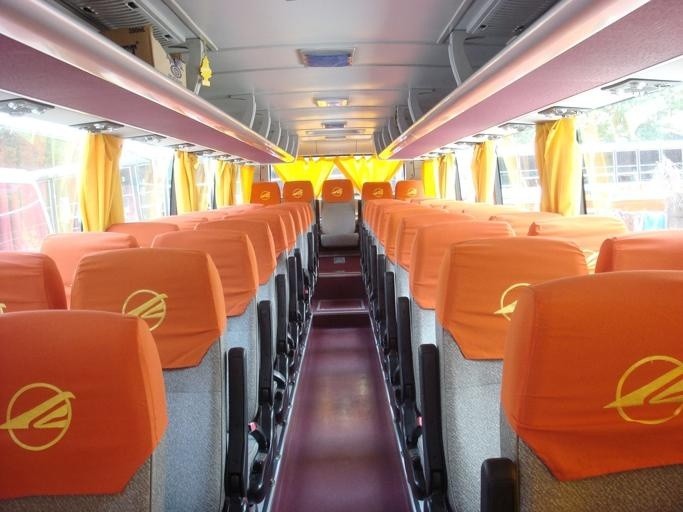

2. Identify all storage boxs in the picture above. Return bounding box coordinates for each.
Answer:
[99,27,187,86]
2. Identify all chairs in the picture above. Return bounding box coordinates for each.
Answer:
[318,179,359,247]
[249,182,282,204]
[283,181,313,200]
[361,182,394,198]
[360,198,682,512]
[395,181,426,199]
[167,202,318,385]
[0,220,320,512]
